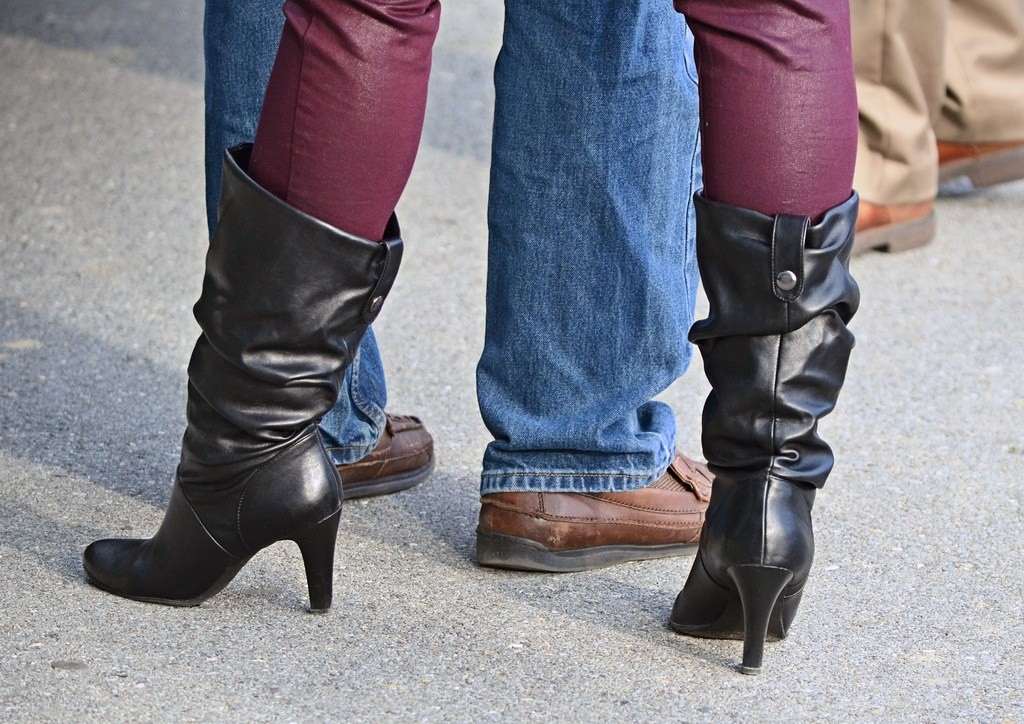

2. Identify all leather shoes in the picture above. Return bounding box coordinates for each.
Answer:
[336,414,434,499]
[852,198,936,257]
[937,140,1024,188]
[477,450,716,571]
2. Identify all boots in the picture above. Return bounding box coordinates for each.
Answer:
[82,142,404,614]
[669,188,860,675]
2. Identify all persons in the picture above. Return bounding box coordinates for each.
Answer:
[82,1,862,677]
[849,0,1023,261]
[196,1,720,575]
